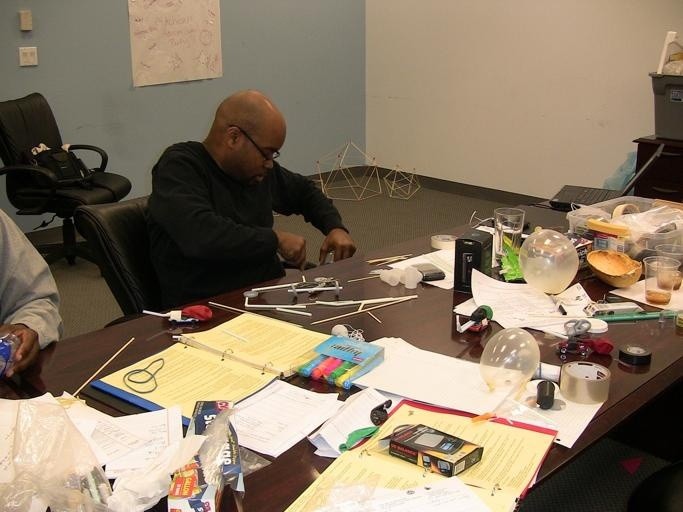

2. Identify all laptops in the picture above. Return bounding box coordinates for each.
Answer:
[549,143,665,209]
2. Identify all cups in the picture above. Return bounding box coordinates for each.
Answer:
[655,243,682,290]
[493,206,525,261]
[643,256,681,306]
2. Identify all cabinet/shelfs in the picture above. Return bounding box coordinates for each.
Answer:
[632,135,683,203]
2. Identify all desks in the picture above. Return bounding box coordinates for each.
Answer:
[0,205,683,512]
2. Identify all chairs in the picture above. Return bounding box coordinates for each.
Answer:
[74,193,163,329]
[1,92,132,278]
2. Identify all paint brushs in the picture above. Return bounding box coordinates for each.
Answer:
[300,354,363,387]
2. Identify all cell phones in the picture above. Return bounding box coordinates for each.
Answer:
[479,218,531,232]
[413,263,445,281]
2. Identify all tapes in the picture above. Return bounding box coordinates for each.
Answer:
[560,361,611,405]
[612,204,640,218]
[619,345,652,366]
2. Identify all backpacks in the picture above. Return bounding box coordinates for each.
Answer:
[32,142,96,187]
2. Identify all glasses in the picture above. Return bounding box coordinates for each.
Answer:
[228,124,280,161]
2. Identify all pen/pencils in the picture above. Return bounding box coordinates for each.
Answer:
[550,294,566,315]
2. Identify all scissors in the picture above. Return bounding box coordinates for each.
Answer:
[564,319,591,338]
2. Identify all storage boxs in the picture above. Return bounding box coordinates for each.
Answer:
[648,72,683,141]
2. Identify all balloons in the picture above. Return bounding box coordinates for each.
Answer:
[480,328,541,392]
[519,229,579,295]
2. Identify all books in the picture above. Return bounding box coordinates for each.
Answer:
[283,400,558,512]
[99,311,333,419]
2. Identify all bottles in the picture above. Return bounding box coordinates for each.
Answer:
[0,333,21,375]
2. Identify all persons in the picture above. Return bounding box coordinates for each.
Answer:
[148,88,357,308]
[0,208,64,380]
